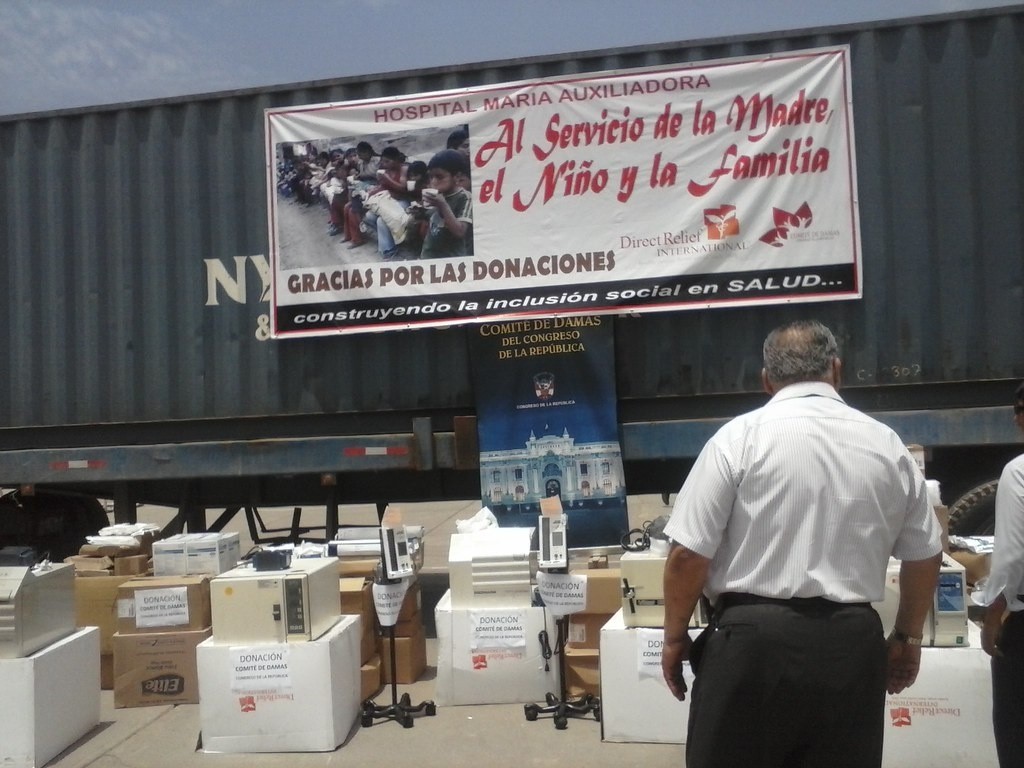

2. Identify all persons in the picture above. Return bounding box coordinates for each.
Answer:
[663,320,943,768]
[970,381,1024,768]
[277,130,473,260]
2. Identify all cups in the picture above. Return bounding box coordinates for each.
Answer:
[422,188,438,209]
[407,180,417,191]
[347,176,354,184]
[377,170,386,174]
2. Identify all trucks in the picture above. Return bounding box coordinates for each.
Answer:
[0,1,1024,548]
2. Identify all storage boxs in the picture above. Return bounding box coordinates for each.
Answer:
[0,444,1004,767]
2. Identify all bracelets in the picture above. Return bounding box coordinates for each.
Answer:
[892,626,923,645]
